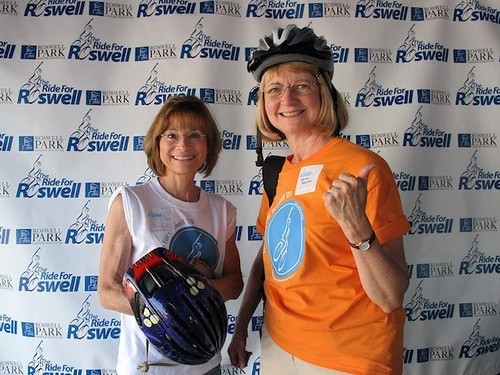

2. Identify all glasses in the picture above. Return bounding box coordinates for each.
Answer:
[258,70,321,99]
[160,130,207,144]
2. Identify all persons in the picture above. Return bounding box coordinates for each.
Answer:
[98,96,244,375]
[225,24,413,375]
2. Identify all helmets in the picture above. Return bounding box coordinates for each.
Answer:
[120,246,229,366]
[248,24,333,83]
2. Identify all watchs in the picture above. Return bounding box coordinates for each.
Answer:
[347,230,378,253]
